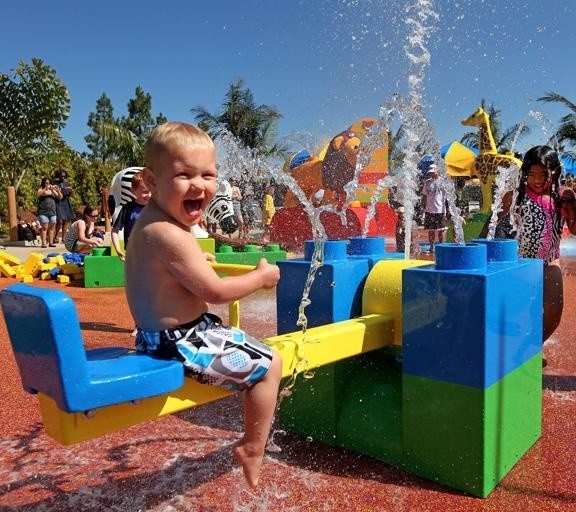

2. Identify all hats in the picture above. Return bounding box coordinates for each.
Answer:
[427,164,439,173]
[396,207,405,213]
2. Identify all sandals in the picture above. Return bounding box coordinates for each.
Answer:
[55,235,66,242]
[41,244,57,248]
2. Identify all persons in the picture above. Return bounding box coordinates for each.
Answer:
[125,123,283,493]
[420,165,469,254]
[48,170,73,243]
[559,172,576,195]
[477,145,576,367]
[227,174,276,239]
[111,170,153,262]
[36,176,64,248]
[63,205,103,254]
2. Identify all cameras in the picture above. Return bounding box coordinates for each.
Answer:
[50,185,54,190]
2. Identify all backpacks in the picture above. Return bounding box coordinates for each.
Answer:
[18,222,36,241]
